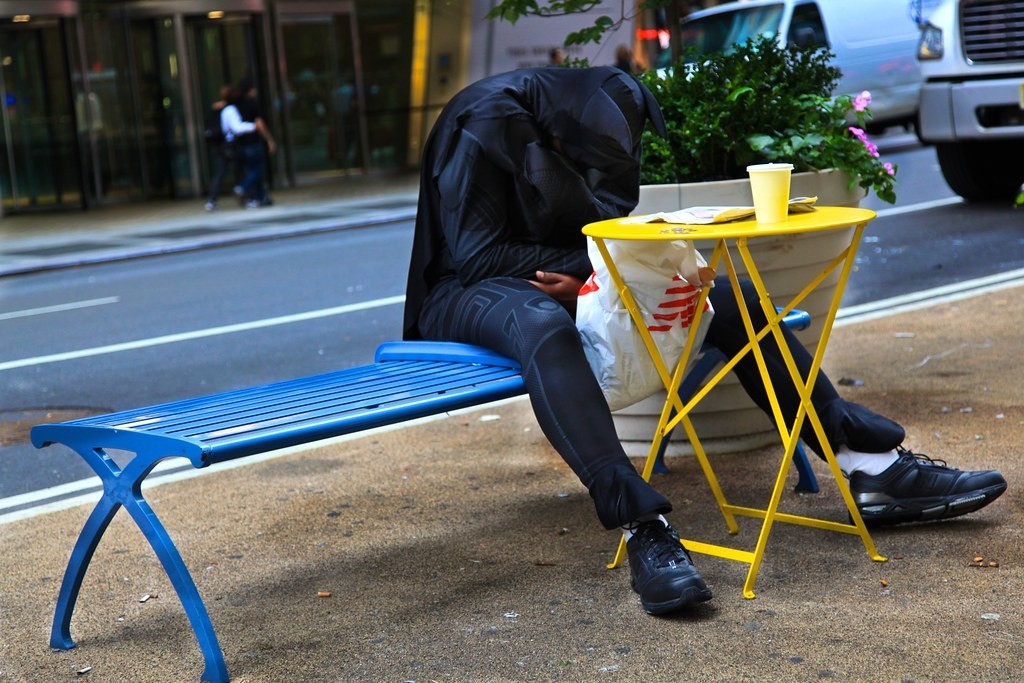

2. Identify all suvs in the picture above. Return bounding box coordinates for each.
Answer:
[908,1,1024,206]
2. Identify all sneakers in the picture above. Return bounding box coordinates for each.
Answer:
[615,495,712,616]
[839,445,1008,526]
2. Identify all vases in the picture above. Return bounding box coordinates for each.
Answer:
[612,169,867,456]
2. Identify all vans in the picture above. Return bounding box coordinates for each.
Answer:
[637,0,941,149]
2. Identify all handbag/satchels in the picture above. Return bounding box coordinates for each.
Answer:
[576,235,715,412]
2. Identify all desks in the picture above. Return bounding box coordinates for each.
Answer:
[581,205,886,598]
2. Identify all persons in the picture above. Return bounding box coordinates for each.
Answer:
[613,43,644,75]
[205,79,277,211]
[549,46,565,67]
[404,67,1007,614]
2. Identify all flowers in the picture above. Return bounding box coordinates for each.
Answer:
[483,0,899,208]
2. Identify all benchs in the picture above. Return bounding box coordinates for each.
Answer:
[28,306,811,682]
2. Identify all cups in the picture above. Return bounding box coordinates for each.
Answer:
[746,163,795,225]
